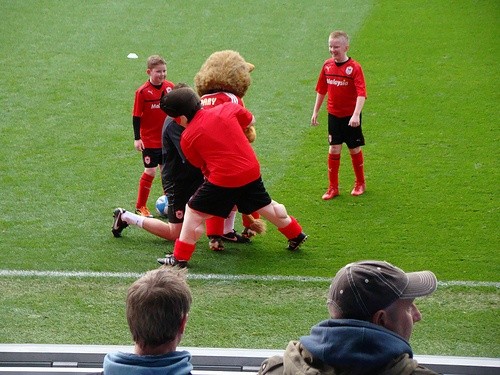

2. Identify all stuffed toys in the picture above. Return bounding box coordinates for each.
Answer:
[194,49,264,249]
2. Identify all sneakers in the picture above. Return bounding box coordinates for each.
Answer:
[135,207,153,218]
[222,229,251,243]
[322,187,338,200]
[112,208,128,238]
[351,182,366,196]
[287,232,307,251]
[157,256,187,268]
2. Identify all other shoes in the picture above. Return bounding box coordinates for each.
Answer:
[242,228,257,239]
[209,237,224,251]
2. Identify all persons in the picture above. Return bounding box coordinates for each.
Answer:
[311,30,367,200]
[106,265,193,375]
[133,54,175,216]
[257,261,437,375]
[110,82,247,244]
[157,86,310,270]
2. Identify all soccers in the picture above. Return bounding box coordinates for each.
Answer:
[155,195,168,217]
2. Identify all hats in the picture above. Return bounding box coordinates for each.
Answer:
[327,260,437,322]
